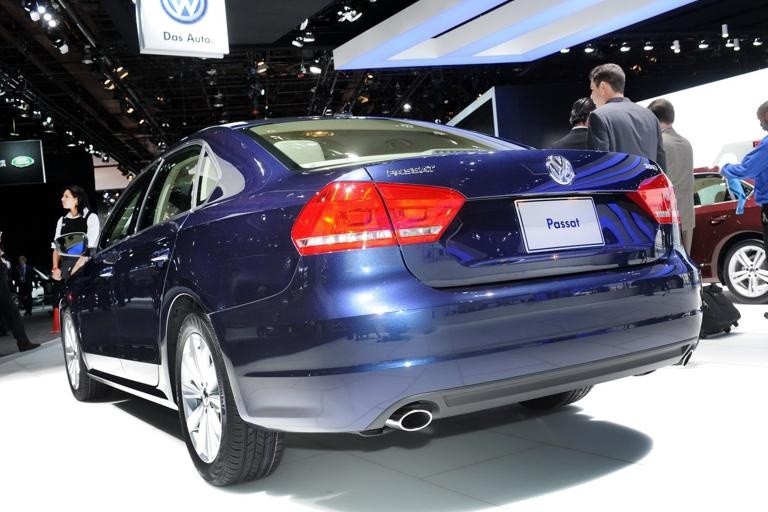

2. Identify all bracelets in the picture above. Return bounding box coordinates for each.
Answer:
[51,267,59,270]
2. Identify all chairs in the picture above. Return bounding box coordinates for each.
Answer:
[273,139,325,165]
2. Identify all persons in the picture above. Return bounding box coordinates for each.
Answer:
[720,102,768,319]
[552,97,596,148]
[648,99,695,258]
[585,62,667,174]
[13,256,33,315]
[51,186,100,285]
[0,259,40,352]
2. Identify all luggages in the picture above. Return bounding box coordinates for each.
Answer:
[699,282,741,339]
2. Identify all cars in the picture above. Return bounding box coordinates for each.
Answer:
[689,165,768,305]
[54,114,707,488]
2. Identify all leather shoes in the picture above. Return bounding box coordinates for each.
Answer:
[19,342,40,352]
